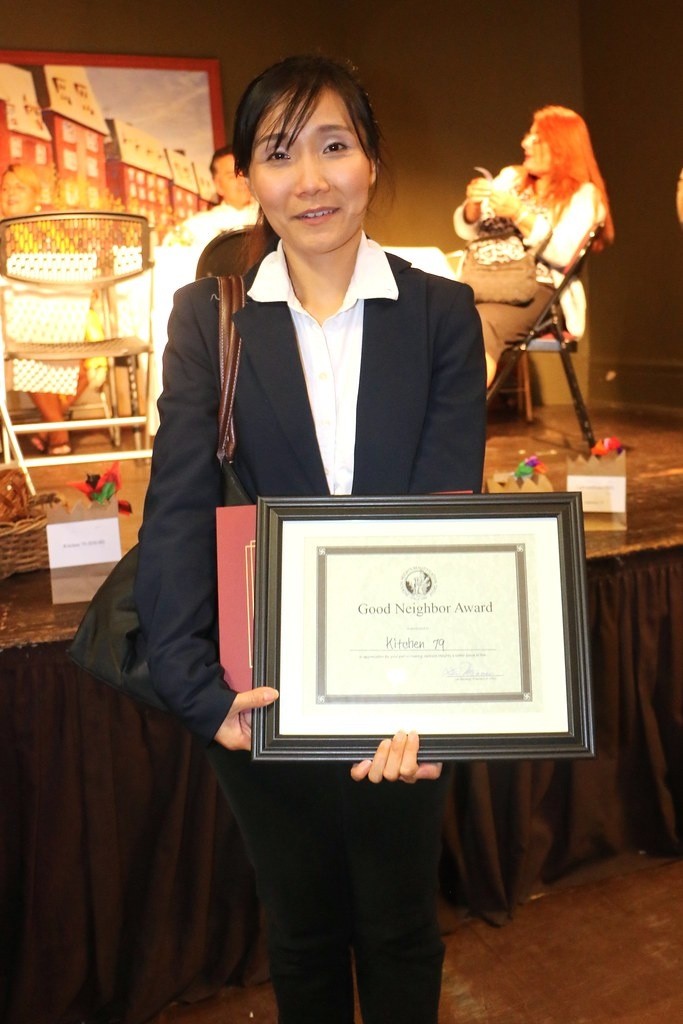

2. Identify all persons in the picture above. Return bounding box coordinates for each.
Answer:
[136,57,487,1023]
[164,145,261,254]
[451,104,615,388]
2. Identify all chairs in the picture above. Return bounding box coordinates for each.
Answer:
[475,220,606,445]
[1,210,159,496]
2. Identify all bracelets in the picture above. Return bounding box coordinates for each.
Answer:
[515,210,529,226]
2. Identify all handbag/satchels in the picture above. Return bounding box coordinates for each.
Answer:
[459,250,536,305]
[66,273,255,713]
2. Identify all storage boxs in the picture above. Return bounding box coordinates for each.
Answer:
[565,451,629,516]
[42,503,123,569]
[484,475,553,494]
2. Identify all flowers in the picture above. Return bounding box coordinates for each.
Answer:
[592,434,623,455]
[514,456,548,477]
[67,465,135,518]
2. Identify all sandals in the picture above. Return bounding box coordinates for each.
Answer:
[47,436,72,456]
[29,432,46,453]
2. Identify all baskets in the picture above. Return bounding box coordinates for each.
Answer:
[0,493,70,578]
